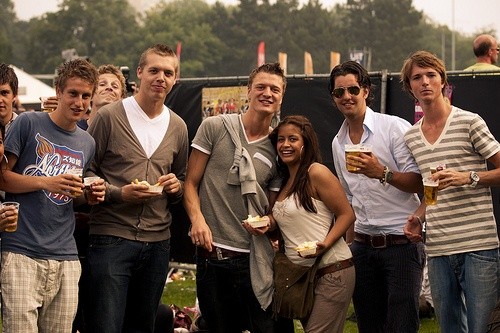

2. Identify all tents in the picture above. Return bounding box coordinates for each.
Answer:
[7,64,57,104]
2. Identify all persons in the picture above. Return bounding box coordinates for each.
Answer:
[85,45,189,333]
[0,59,105,333]
[76,64,126,131]
[328,61,424,333]
[241,116,357,333]
[399,51,500,333]
[183,63,294,333]
[202,99,250,117]
[459,34,500,77]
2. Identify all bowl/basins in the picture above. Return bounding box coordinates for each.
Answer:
[243,220,269,228]
[132,182,164,193]
[298,245,317,257]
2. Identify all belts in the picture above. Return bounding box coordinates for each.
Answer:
[317,257,354,278]
[353,232,408,249]
[196,246,244,261]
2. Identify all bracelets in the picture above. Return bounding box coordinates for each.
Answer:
[382,166,388,185]
[414,215,422,226]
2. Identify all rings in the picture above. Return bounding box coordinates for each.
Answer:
[196,239,199,241]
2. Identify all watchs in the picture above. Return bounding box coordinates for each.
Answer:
[470,171,480,186]
[386,170,394,183]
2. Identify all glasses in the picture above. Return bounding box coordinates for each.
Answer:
[487,47,499,54]
[331,86,363,98]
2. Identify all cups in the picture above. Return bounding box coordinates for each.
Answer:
[345,144,372,172]
[67,168,83,196]
[430,163,447,187]
[423,177,439,205]
[84,176,101,205]
[2,202,20,232]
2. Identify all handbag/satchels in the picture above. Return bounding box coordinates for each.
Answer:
[168,303,192,328]
[271,251,317,322]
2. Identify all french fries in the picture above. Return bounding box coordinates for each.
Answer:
[134,178,161,186]
[294,246,311,249]
